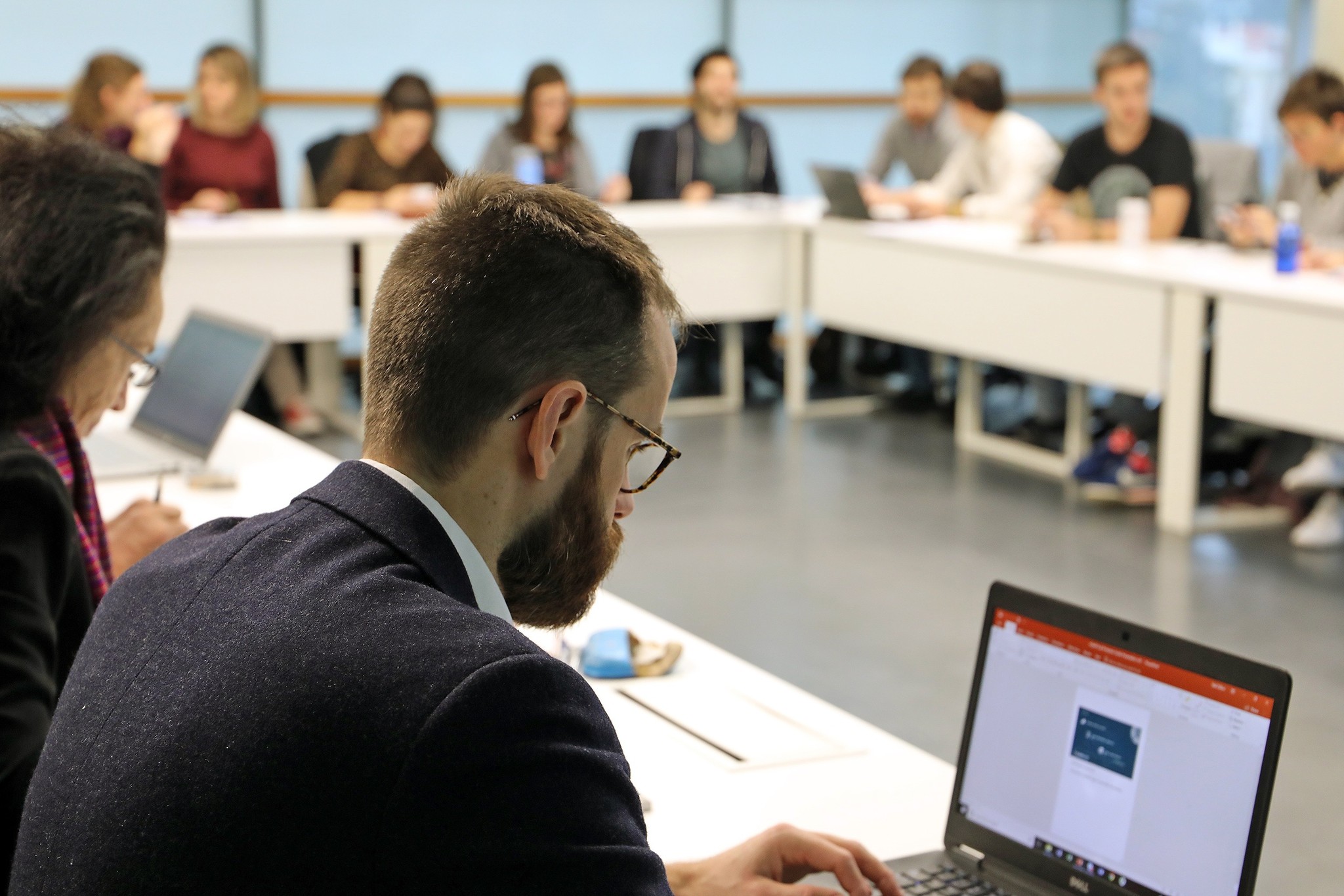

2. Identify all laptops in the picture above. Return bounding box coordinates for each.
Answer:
[81,312,274,479]
[813,166,905,221]
[794,581,1292,896]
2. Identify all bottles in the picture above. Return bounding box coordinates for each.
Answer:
[1274,199,1302,271]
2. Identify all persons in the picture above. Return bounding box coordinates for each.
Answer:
[905,62,1065,431]
[0,110,190,896]
[994,38,1204,445]
[854,55,966,411]
[1,170,902,896]
[1073,64,1344,547]
[53,43,809,435]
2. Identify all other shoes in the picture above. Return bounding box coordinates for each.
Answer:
[1282,447,1343,491]
[282,398,326,438]
[1288,491,1344,550]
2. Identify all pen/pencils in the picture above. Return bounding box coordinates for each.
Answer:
[154,471,164,502]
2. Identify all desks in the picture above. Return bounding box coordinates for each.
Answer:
[80,195,1344,896]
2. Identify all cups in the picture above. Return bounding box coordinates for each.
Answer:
[1115,196,1151,247]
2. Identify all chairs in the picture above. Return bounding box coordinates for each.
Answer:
[1199,142,1264,237]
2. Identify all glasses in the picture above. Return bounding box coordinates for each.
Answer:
[508,390,681,493]
[103,331,158,387]
